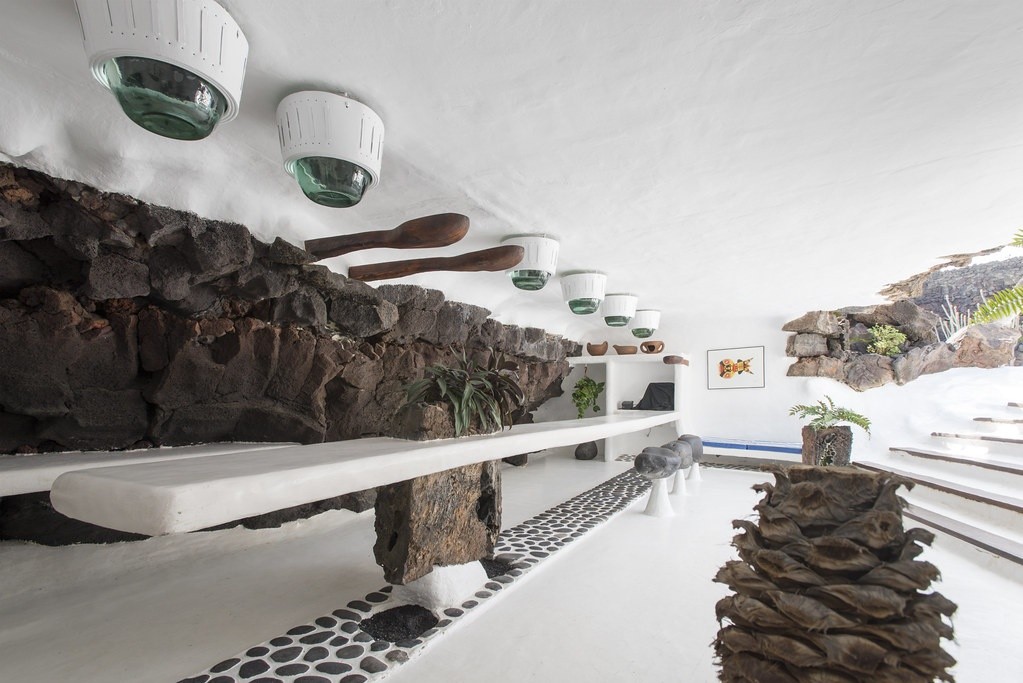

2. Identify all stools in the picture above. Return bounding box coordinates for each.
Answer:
[677,433,706,493]
[663,440,693,496]
[633,447,679,517]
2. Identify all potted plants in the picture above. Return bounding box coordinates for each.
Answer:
[788,396,875,469]
[386,343,526,444]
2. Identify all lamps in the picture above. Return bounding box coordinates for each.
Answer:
[501,235,559,294]
[629,308,662,339]
[557,271,605,314]
[76,0,255,142]
[601,295,638,327]
[274,90,384,210]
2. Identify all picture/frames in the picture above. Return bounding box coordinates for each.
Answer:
[706,345,765,391]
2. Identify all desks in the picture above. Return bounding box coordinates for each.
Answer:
[50,410,677,616]
